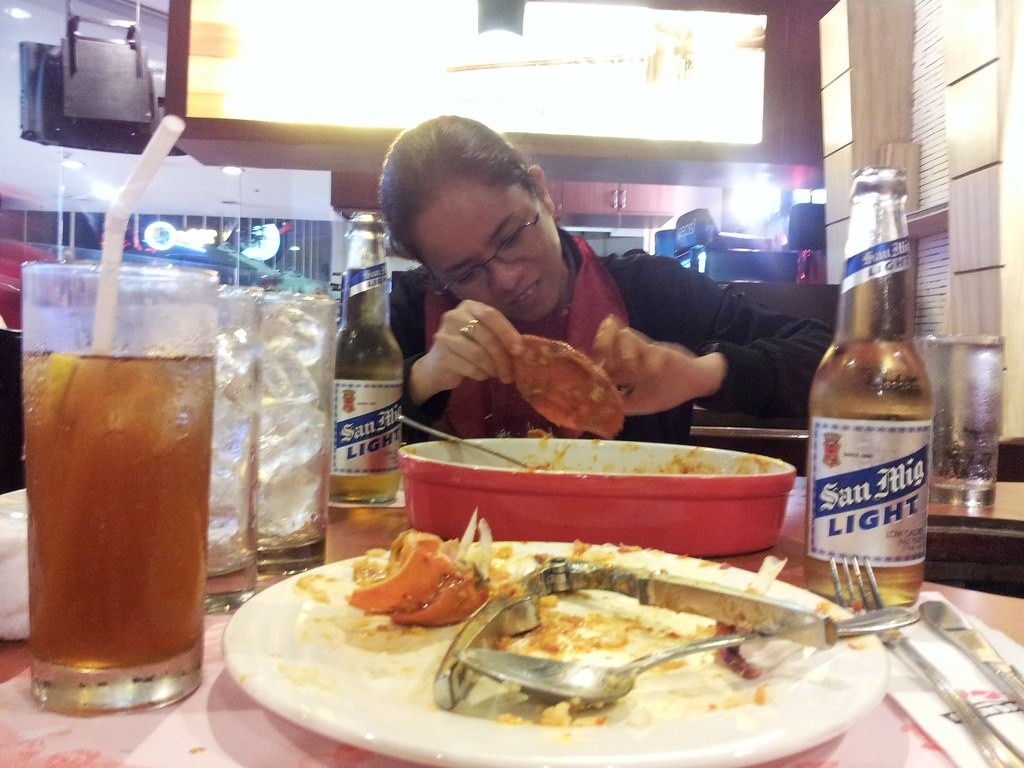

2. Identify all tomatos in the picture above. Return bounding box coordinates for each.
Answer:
[351,529,441,606]
[394,568,487,627]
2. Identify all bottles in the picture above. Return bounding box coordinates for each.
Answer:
[805,166,934,608]
[328,208,402,505]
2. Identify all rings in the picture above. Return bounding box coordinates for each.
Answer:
[459,319,479,337]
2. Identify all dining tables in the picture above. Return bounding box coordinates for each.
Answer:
[0,472,1024,768]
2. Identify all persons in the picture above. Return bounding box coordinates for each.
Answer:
[376,114,834,447]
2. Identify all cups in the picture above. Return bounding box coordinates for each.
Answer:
[909,334,1006,506]
[18,258,218,717]
[198,286,266,616]
[257,292,339,573]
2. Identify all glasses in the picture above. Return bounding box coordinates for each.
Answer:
[429,186,540,299]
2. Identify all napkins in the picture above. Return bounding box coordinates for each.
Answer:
[884,589,1024,768]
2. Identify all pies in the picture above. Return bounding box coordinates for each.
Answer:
[514,334,624,434]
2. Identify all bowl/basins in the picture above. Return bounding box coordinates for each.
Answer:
[398,438,797,558]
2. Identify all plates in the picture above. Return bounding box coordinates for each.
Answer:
[221,541,893,768]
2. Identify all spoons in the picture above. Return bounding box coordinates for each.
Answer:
[458,606,923,709]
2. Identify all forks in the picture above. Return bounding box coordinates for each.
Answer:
[830,556,1024,768]
